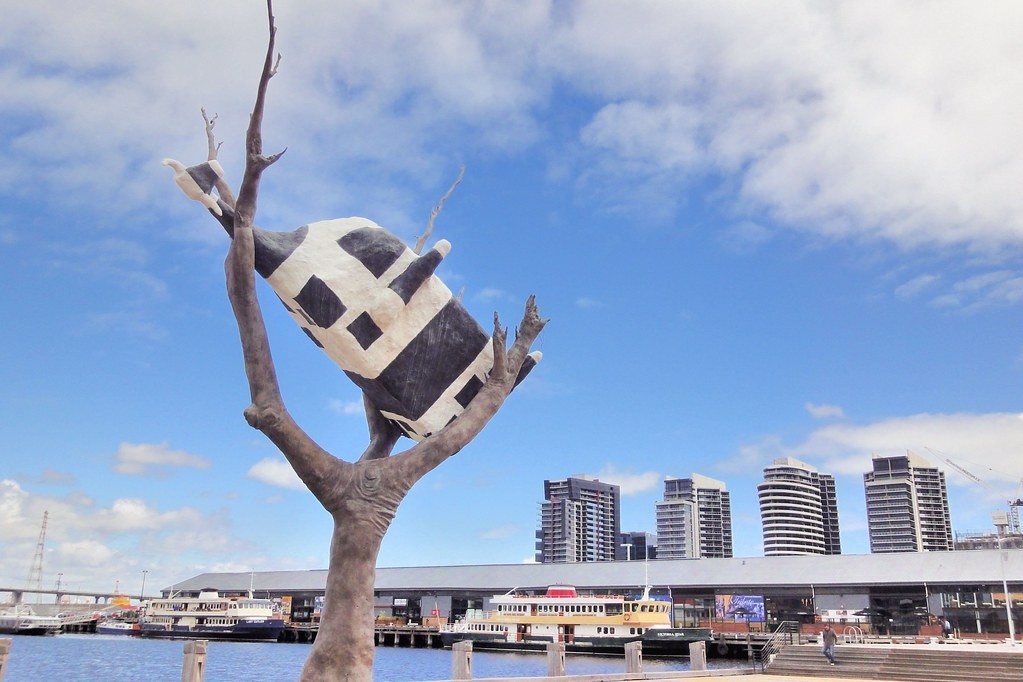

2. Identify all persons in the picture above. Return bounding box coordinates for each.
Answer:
[821,623,838,666]
[938,618,951,638]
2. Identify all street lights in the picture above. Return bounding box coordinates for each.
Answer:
[140,570,148,602]
[58,573,63,600]
[115,580,119,593]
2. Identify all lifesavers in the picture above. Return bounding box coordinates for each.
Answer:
[624,614,631,621]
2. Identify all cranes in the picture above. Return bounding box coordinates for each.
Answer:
[925,445,1023,535]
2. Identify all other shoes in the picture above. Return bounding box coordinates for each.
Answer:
[831,662,834,666]
[828,659,831,664]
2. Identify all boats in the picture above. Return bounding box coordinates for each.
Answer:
[94,617,141,636]
[134,587,290,644]
[434,583,715,659]
[0,604,62,636]
